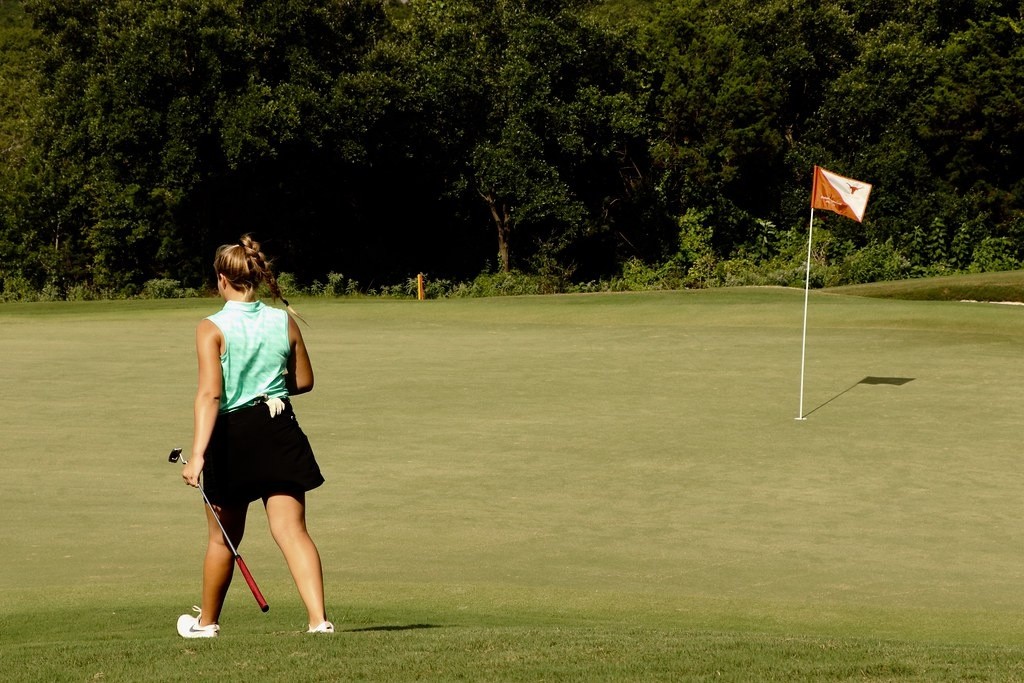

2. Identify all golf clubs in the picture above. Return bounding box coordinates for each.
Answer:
[167,447,270,613]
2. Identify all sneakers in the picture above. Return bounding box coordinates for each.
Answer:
[306,621,334,634]
[176,606,219,639]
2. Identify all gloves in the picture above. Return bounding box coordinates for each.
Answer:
[254,392,285,418]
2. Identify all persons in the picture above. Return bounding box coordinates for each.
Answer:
[177,237,331,637]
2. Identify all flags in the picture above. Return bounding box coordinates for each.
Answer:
[811,166,872,223]
